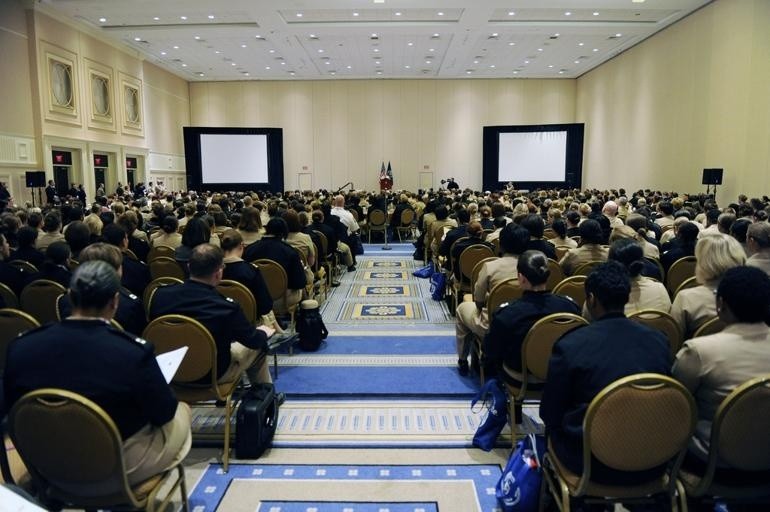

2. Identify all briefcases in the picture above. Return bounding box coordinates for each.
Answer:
[235,383,278,459]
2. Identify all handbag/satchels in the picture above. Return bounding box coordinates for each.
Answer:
[429,272,447,301]
[411,260,434,278]
[295,314,328,353]
[494,433,542,512]
[470,378,509,452]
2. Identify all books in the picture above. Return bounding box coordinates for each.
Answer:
[151,345,190,388]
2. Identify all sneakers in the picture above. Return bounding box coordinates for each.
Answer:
[348,265,356,272]
[457,359,469,376]
[277,391,286,407]
[265,329,300,351]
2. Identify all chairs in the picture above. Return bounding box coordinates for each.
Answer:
[364,203,479,316]
[479,205,770,512]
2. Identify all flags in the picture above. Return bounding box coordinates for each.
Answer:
[379,161,390,180]
[386,161,393,179]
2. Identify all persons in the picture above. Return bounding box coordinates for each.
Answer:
[1,178,357,341]
[670,266,770,511]
[345,189,428,239]
[148,242,285,405]
[479,249,581,424]
[419,177,770,340]
[456,222,533,369]
[1,260,192,491]
[539,258,670,511]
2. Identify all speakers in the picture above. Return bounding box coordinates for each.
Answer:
[25,171,46,187]
[701,168,723,185]
